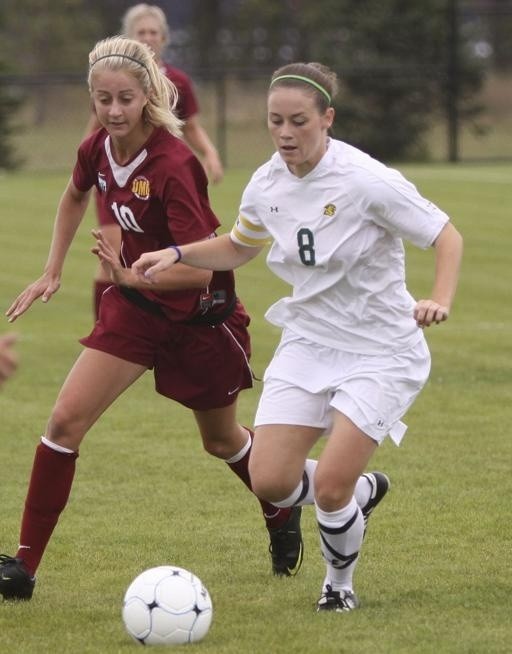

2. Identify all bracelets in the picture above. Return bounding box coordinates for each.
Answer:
[168,246,181,263]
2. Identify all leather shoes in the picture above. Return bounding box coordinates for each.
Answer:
[267,506,303,576]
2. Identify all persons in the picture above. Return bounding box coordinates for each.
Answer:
[0,37,303,603]
[131,62,463,616]
[80,5,224,325]
[0,328,20,387]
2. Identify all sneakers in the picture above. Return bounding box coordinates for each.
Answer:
[361,472,391,540]
[317,585,359,613]
[0,554,35,599]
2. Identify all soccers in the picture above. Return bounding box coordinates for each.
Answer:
[122,566,212,644]
